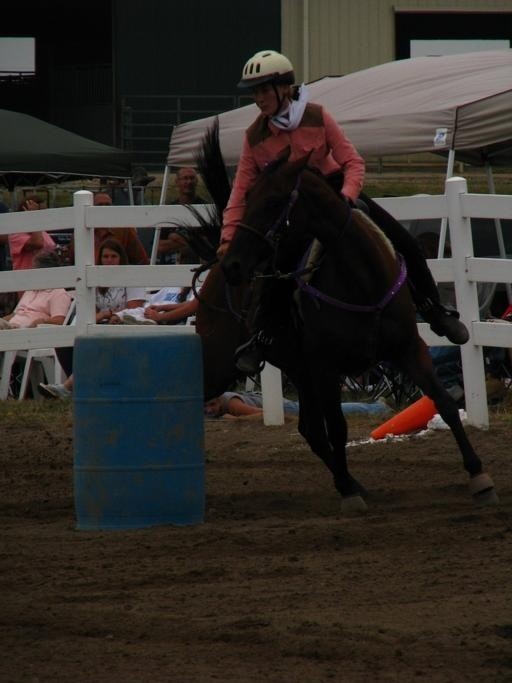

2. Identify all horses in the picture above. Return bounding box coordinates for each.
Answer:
[192,113,502,521]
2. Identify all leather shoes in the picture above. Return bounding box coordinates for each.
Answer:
[232,338,265,375]
[418,303,471,347]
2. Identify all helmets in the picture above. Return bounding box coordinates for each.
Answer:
[234,47,297,91]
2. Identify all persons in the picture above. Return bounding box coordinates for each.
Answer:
[203,392,392,419]
[344,232,511,411]
[1,168,208,403]
[216,49,468,344]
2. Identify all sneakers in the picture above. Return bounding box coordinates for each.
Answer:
[37,382,72,400]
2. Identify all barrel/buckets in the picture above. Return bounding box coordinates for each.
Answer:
[69,324,206,533]
